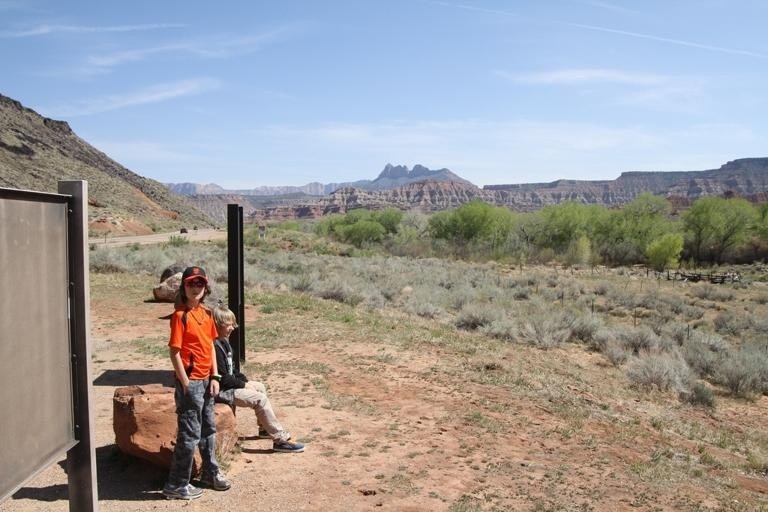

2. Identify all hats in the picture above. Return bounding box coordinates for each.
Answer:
[182,266,208,283]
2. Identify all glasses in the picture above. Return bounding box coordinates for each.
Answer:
[186,281,204,288]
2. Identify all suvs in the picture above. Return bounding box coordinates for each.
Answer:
[180,228,188,233]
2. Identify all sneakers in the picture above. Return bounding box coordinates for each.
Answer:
[259,430,271,439]
[163,483,203,499]
[273,440,305,452]
[200,470,230,490]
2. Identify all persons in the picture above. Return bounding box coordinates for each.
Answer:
[210,305,306,453]
[165,266,231,498]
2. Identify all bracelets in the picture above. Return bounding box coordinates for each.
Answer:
[210,371,226,383]
